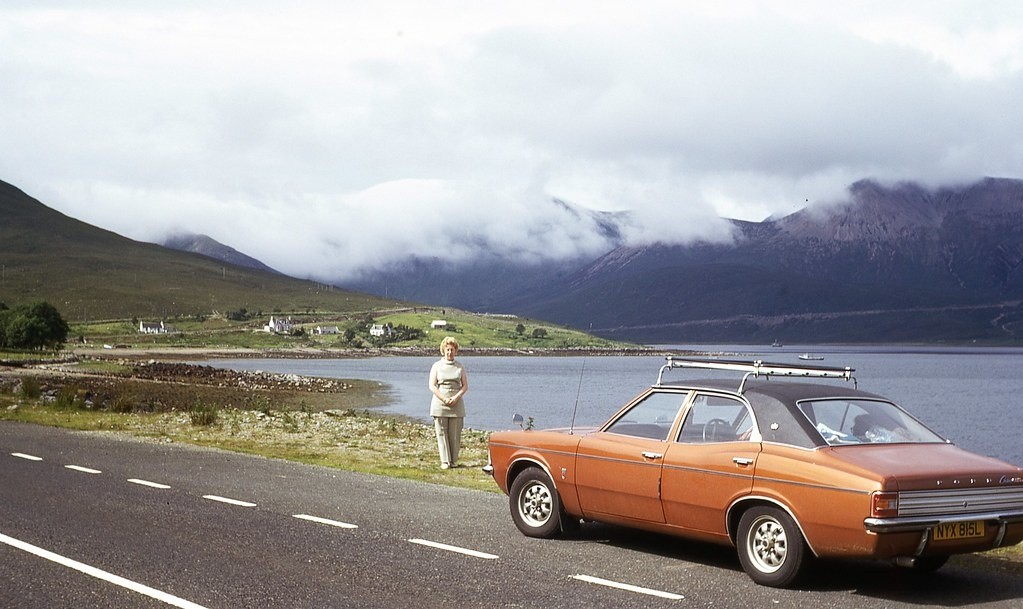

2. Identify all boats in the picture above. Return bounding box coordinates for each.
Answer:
[798,352,824,360]
[772,339,782,347]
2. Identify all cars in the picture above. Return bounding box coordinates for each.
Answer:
[481,357,1023,588]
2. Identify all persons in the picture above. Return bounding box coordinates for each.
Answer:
[428,337,468,469]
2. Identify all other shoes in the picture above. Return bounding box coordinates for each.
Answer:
[440,464,448,469]
[450,463,458,468]
